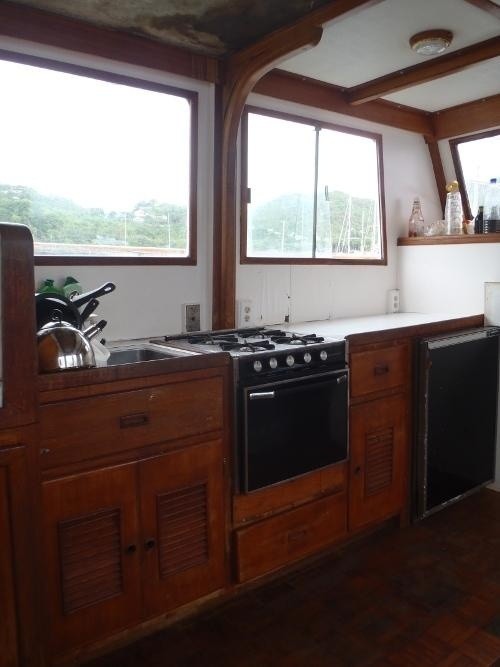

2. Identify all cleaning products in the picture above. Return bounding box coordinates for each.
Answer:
[62,272,84,301]
[38,277,60,297]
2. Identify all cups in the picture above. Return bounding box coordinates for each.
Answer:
[465,222,475,234]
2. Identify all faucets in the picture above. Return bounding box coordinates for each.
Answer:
[84,313,100,326]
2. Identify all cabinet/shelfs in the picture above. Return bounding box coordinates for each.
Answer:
[233,459,350,588]
[350,337,411,532]
[0,223,55,666]
[37,352,230,660]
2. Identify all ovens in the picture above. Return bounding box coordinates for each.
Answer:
[233,367,351,495]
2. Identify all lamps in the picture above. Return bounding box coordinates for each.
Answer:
[408,28,454,56]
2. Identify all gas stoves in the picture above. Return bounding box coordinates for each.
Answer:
[149,323,343,382]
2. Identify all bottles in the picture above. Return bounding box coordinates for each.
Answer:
[474,177,500,233]
[444,181,464,235]
[408,195,425,236]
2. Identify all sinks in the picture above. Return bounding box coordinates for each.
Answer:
[106,343,206,367]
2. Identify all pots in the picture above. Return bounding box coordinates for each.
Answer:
[34,281,118,332]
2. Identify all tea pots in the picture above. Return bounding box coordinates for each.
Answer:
[36,309,109,375]
